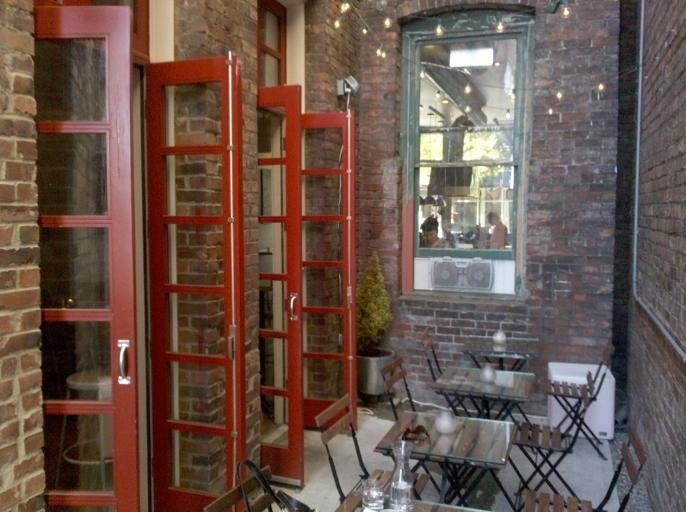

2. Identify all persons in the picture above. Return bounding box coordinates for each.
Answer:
[419,215,452,250]
[486,210,507,249]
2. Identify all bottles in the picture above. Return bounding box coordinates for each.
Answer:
[389,440,415,512]
[435,408,456,434]
[492,329,507,344]
[479,362,496,384]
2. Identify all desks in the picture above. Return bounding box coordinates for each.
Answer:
[335,492,482,512]
[376,410,516,512]
[433,365,536,422]
[456,333,539,373]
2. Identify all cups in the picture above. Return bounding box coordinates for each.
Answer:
[362,478,385,510]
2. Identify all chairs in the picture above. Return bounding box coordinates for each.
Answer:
[315,393,431,503]
[204,463,289,512]
[514,371,594,495]
[523,425,648,512]
[542,340,615,460]
[419,326,481,417]
[379,358,453,490]
